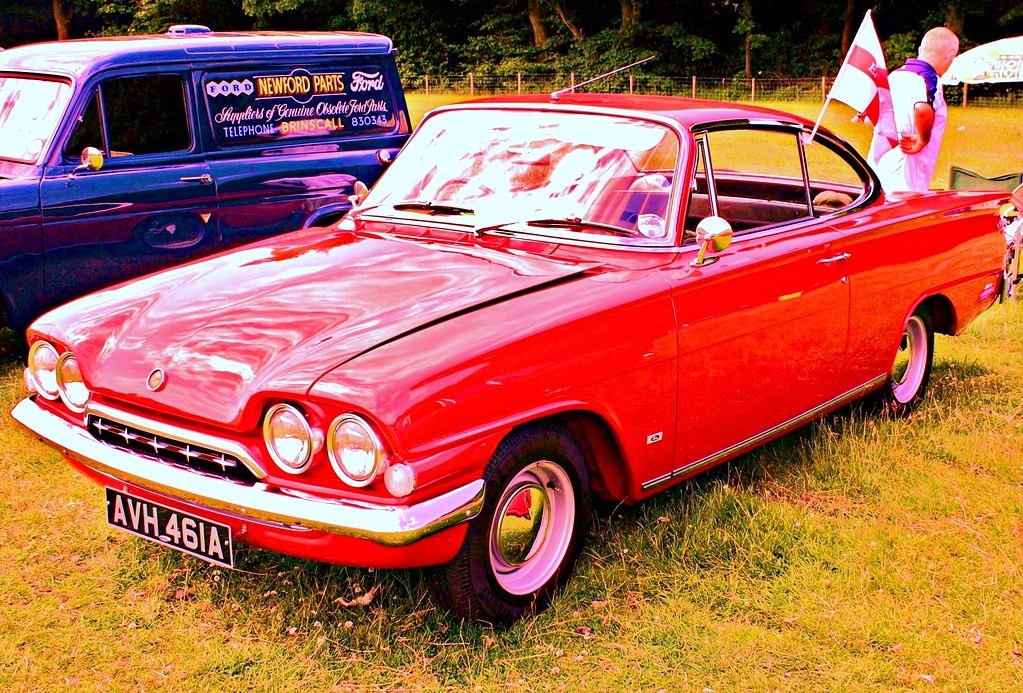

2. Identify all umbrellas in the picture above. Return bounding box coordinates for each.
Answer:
[938,36,1023,86]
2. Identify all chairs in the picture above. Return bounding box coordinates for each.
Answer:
[613,174,672,238]
[948,166,1023,303]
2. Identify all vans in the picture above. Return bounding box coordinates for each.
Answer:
[0,25,412,356]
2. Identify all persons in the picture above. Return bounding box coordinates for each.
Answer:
[865,27,959,194]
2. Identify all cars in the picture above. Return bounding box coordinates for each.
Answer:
[11,91,1023,631]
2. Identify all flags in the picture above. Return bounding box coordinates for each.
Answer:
[827,12,899,166]
